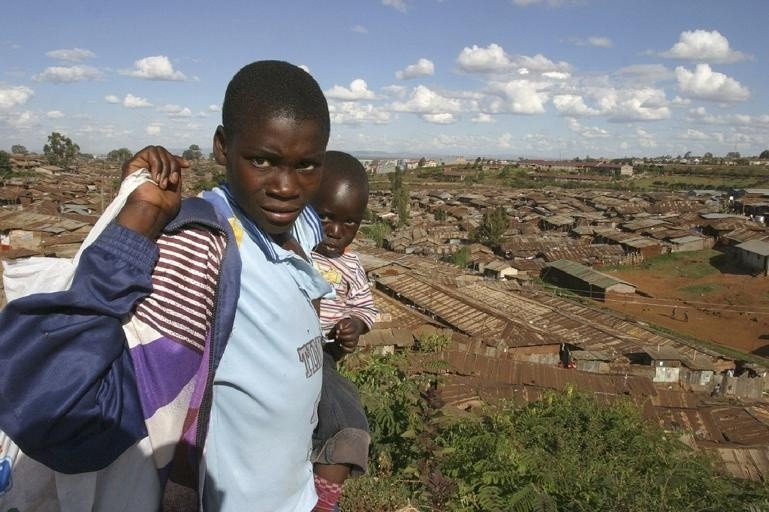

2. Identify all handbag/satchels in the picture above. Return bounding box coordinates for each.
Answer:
[0,168,163,512]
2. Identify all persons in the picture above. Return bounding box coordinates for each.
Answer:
[303,150,379,511]
[1,59,332,512]
